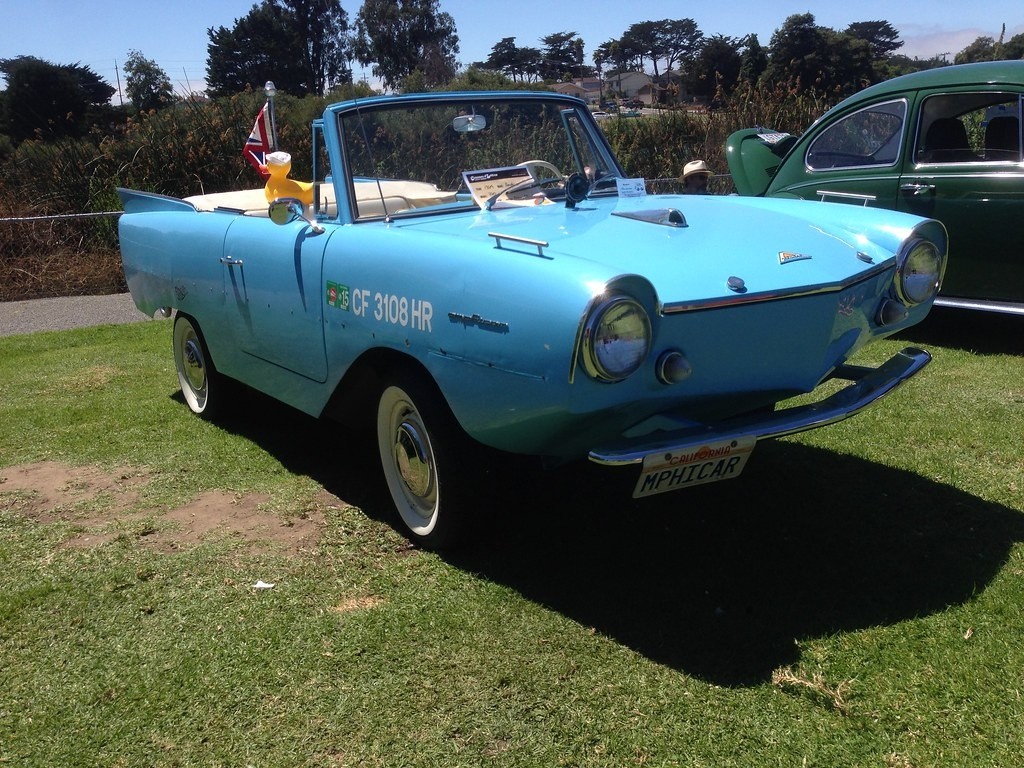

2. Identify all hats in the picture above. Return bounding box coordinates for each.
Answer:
[678,159,715,184]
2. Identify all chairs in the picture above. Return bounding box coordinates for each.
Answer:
[985,116,1020,160]
[924,118,972,162]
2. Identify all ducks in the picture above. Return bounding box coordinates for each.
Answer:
[264,151,314,204]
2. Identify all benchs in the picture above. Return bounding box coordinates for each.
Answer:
[245,196,410,221]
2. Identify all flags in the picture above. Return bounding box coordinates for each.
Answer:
[244,105,274,181]
